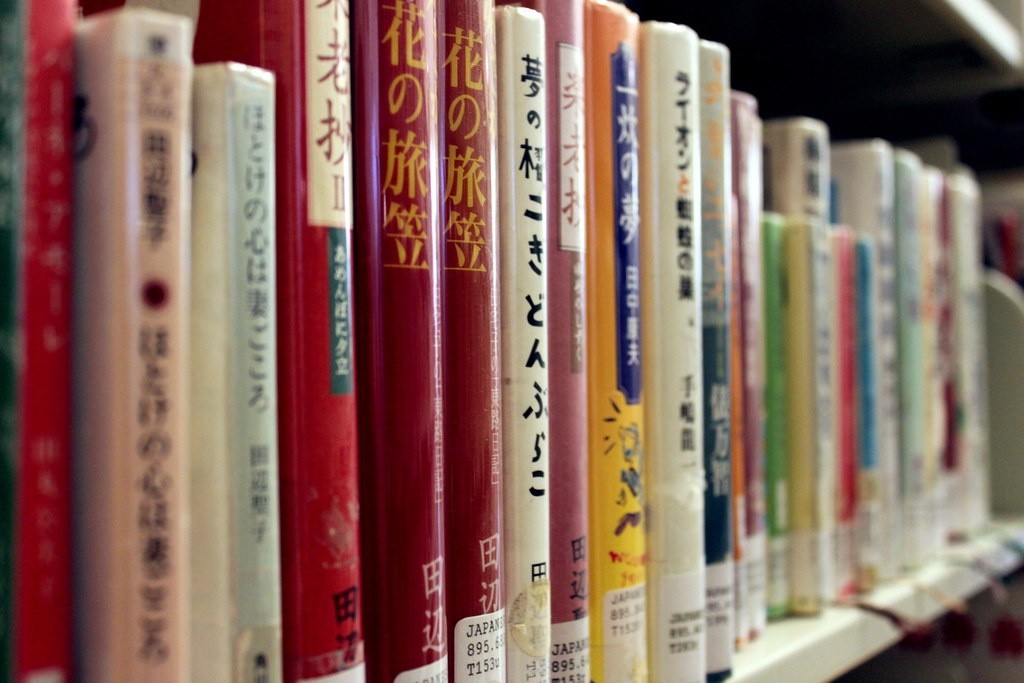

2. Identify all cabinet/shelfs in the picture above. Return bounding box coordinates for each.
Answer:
[729,0,1024,683]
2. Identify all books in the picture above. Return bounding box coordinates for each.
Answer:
[1,0,984,683]
